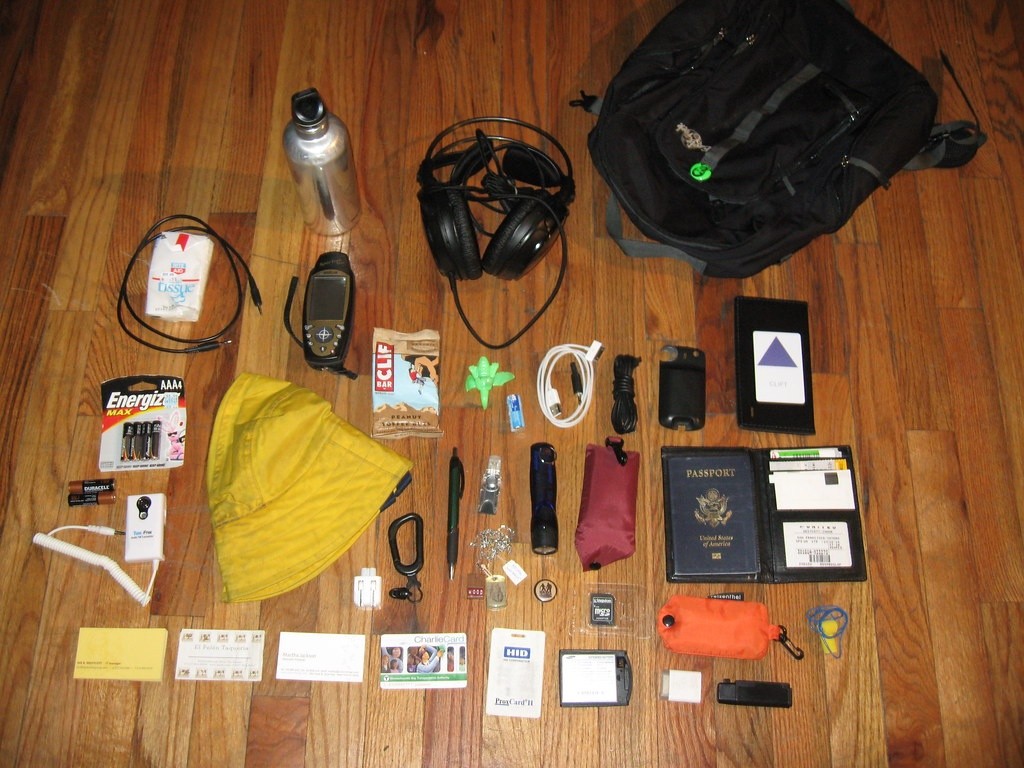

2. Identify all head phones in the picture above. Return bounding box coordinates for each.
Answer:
[417,115,576,281]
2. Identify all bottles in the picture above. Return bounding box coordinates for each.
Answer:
[282,91,361,238]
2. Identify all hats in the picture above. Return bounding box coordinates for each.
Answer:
[207,374,415,603]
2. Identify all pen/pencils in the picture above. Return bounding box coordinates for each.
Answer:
[446,444,466,580]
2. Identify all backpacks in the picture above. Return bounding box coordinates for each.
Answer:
[569,0,987,278]
